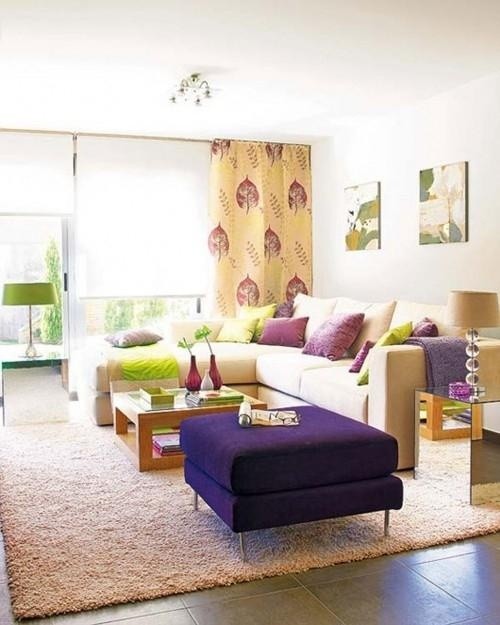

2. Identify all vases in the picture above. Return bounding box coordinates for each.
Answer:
[207,354,223,391]
[198,368,214,393]
[184,355,202,392]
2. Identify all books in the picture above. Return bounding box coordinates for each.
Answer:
[190,389,244,401]
[187,395,244,406]
[152,441,183,452]
[152,446,185,456]
[153,434,181,449]
[238,401,300,428]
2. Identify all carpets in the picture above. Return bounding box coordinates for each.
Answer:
[3,367,498,624]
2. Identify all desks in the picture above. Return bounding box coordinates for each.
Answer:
[411,383,500,506]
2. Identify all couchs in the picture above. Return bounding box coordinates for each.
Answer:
[75,296,500,473]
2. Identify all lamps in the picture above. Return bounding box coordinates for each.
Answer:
[165,71,220,108]
[1,282,60,359]
[440,286,499,394]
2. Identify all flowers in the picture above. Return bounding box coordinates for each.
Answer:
[175,335,197,358]
[194,324,216,355]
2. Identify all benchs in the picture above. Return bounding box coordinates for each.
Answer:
[175,401,406,564]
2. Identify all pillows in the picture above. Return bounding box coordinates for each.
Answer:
[170,291,438,387]
[104,328,164,349]
